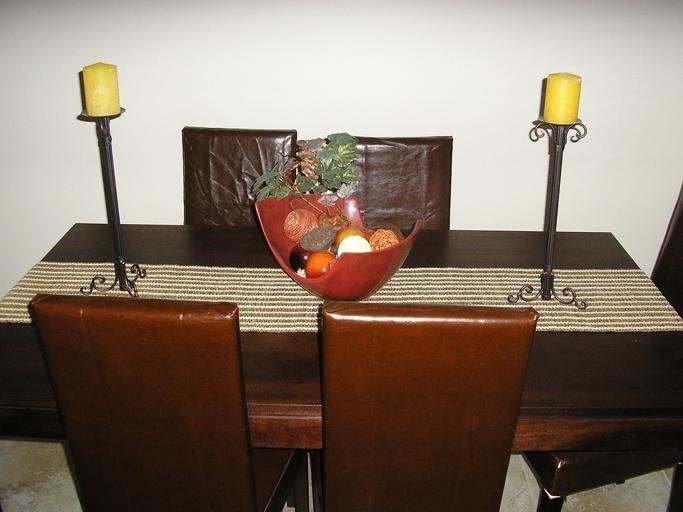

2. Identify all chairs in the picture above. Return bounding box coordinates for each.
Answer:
[326,136,453,232]
[309,299,539,512]
[521,182,683,512]
[182,127,296,227]
[28,293,309,512]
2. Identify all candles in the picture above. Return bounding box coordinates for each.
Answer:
[543,72,582,125]
[82,62,120,117]
